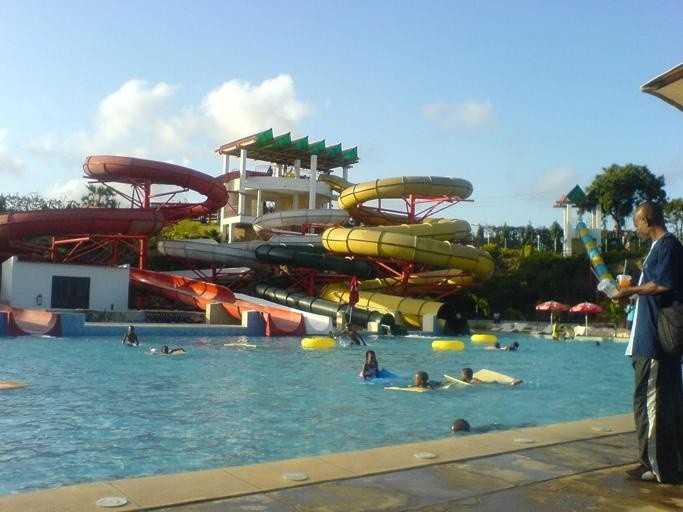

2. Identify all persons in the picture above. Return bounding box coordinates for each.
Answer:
[157,346,185,354]
[358,350,379,381]
[508,342,519,350]
[451,418,470,434]
[122,325,140,347]
[409,371,435,391]
[460,367,483,387]
[550,318,575,342]
[484,342,500,350]
[286,168,295,178]
[608,200,683,485]
[267,164,274,176]
[334,322,366,347]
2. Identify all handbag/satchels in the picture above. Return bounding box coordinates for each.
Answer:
[657,304,683,355]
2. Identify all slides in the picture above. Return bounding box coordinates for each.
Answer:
[1,304,63,339]
[157,209,360,336]
[0,156,308,335]
[317,173,496,337]
[249,242,409,336]
[577,222,616,283]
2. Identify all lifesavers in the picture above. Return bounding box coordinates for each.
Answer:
[432,340,465,351]
[301,339,335,348]
[471,335,498,342]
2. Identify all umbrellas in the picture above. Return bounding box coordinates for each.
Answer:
[569,301,603,336]
[535,299,571,333]
[347,276,361,323]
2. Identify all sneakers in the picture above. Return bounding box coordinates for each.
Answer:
[625,464,656,480]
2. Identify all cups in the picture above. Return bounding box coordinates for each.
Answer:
[596,278,620,299]
[617,274,634,288]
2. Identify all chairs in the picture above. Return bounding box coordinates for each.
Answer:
[491,322,592,335]
